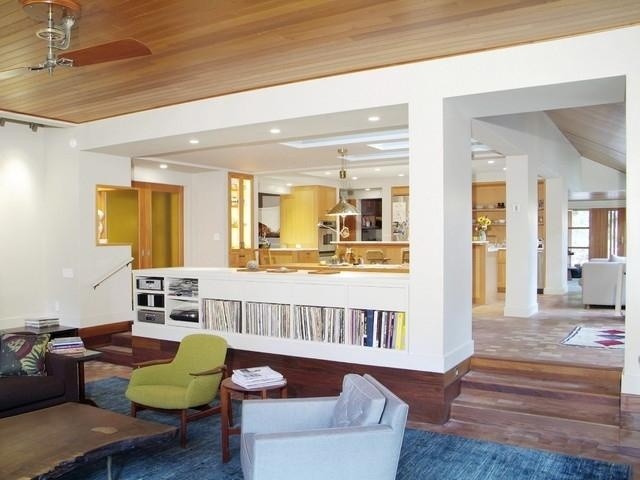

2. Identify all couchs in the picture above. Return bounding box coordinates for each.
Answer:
[579,255,626,310]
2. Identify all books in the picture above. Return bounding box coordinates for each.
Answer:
[230,366,287,390]
[24,318,59,329]
[47,337,86,354]
[203,300,405,350]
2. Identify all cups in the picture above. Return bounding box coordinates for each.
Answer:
[328,256,353,263]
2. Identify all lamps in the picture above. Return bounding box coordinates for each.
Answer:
[327,148,360,216]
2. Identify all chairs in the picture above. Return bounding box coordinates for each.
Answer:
[0,331,66,418]
[367,250,409,264]
[241,373,409,480]
[124,333,233,447]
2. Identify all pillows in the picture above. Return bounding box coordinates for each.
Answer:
[609,254,626,263]
[0,332,51,377]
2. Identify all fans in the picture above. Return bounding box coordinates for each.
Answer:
[0,0,152,79]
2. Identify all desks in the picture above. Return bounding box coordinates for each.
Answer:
[220,377,287,463]
[0,326,103,407]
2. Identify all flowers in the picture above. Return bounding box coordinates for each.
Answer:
[478,216,490,230]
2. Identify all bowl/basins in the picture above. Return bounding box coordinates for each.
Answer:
[319,261,327,266]
[475,202,505,209]
[498,219,505,224]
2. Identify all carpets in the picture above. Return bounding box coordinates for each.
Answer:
[59,375,630,480]
[561,325,626,353]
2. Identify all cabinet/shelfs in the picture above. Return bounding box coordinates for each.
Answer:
[228,171,254,268]
[132,270,412,370]
[472,181,546,248]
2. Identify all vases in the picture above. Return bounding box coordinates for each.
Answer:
[480,229,486,241]
